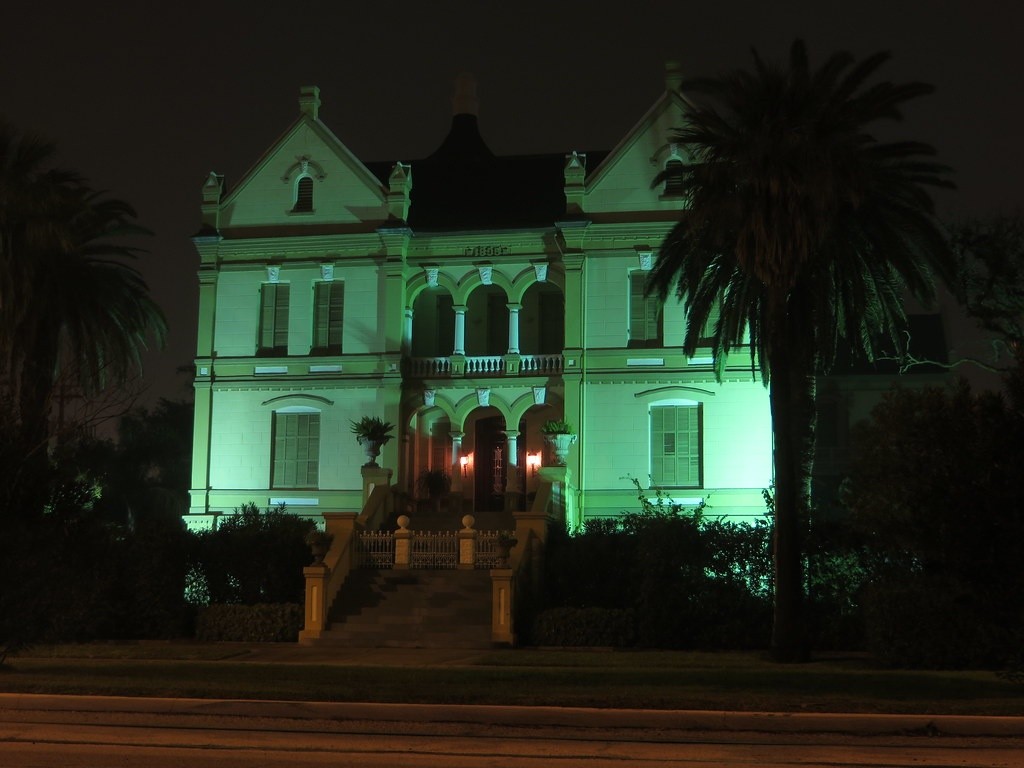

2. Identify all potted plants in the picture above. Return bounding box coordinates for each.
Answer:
[350,417,398,470]
[540,417,577,467]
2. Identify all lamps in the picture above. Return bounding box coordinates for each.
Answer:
[460,449,469,478]
[528,448,540,478]
